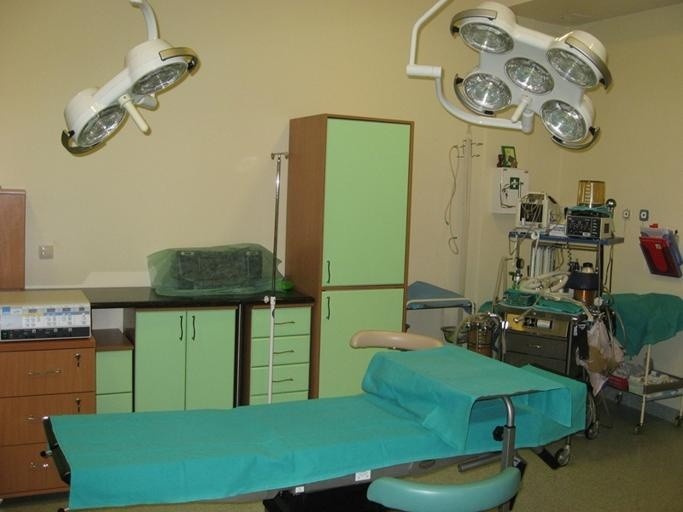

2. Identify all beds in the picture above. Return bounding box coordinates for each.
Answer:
[43,329,588,511]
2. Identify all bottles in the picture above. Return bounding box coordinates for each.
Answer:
[580,262,595,274]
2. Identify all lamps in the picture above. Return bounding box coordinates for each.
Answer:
[404,0,611,151]
[61,0,200,155]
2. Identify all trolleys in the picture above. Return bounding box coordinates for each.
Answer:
[502,302,601,467]
[595,309,683,435]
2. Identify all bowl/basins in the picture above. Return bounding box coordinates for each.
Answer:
[441,326,467,344]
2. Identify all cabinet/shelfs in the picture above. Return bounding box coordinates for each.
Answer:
[246,303,311,404]
[498,303,586,383]
[135,303,238,412]
[285,113,416,399]
[598,293,682,432]
[0,339,97,500]
[94,343,135,417]
[509,230,625,305]
